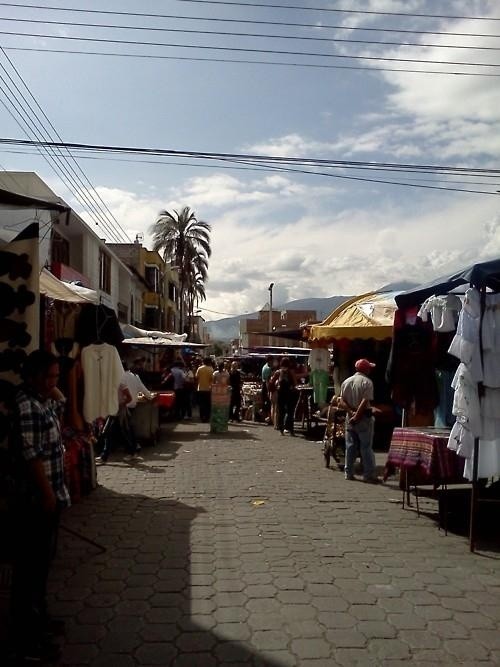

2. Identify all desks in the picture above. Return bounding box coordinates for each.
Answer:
[393,426,465,517]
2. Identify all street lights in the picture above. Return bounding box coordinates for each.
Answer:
[267,281,275,346]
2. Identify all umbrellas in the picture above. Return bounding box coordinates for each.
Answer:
[120,333,211,389]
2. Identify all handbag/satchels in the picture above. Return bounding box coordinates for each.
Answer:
[274,375,289,392]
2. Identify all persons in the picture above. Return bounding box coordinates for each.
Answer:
[94,381,138,462]
[339,357,384,484]
[6,346,70,648]
[122,359,155,451]
[160,350,299,438]
[129,356,152,389]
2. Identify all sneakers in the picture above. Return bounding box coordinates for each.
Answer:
[123,452,144,463]
[183,416,192,420]
[94,457,105,466]
[365,477,384,484]
[344,472,352,480]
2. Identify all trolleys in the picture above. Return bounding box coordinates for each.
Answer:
[321,394,347,469]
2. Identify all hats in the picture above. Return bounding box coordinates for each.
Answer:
[355,359,376,371]
